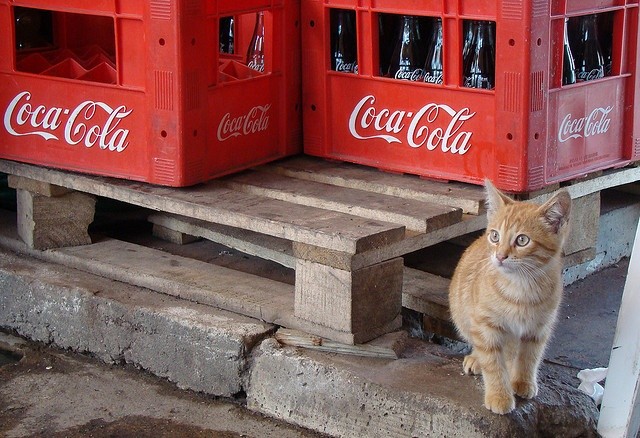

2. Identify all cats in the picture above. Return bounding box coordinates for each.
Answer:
[447,175,572,417]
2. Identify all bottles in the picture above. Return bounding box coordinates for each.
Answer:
[464,21,493,93]
[423,18,445,85]
[388,15,422,83]
[246,10,267,74]
[331,10,360,74]
[580,15,609,84]
[564,17,578,86]
